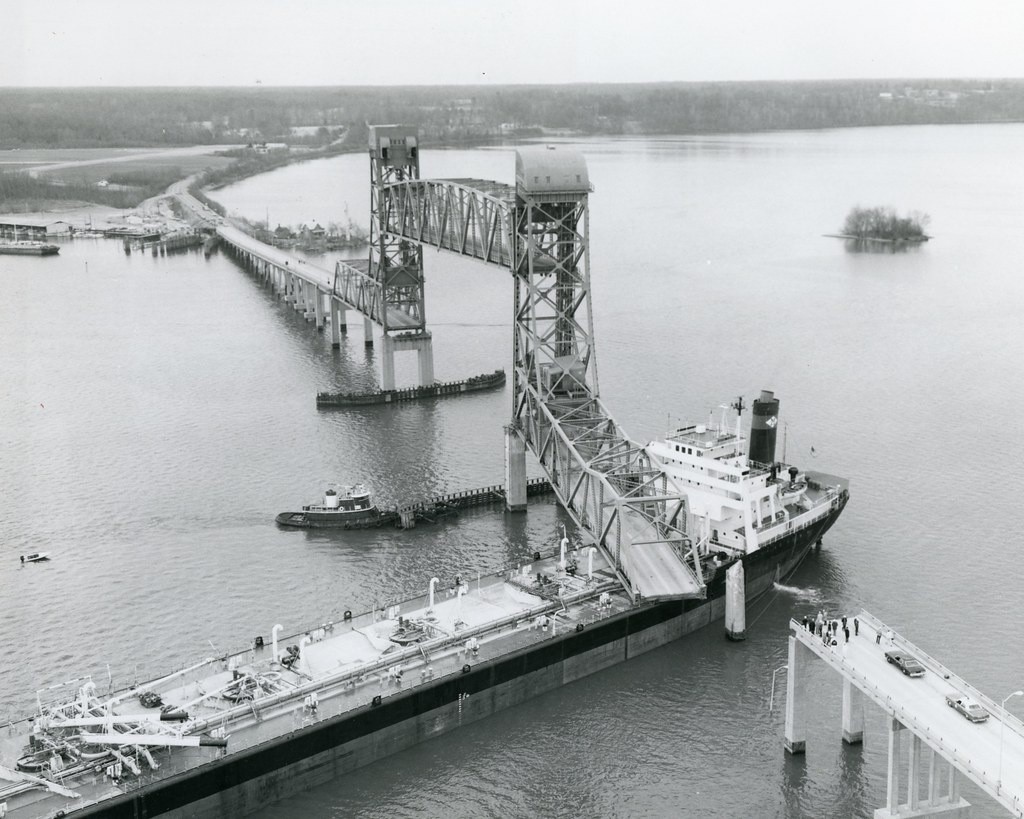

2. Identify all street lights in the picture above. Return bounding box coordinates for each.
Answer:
[998,690,1024,763]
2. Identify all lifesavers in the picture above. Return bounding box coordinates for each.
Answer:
[354,523,361,529]
[344,523,350,530]
[363,522,370,529]
[375,520,382,527]
[339,507,344,511]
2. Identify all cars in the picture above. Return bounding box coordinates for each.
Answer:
[944,689,991,724]
[883,648,927,679]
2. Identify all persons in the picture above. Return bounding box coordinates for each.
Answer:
[853,617,859,636]
[875,627,883,645]
[886,630,894,647]
[841,615,847,631]
[845,627,849,642]
[801,609,838,645]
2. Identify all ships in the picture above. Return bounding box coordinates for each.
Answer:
[0,384,856,819]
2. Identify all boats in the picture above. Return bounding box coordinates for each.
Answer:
[273,481,401,529]
[0,239,61,256]
[18,550,49,564]
